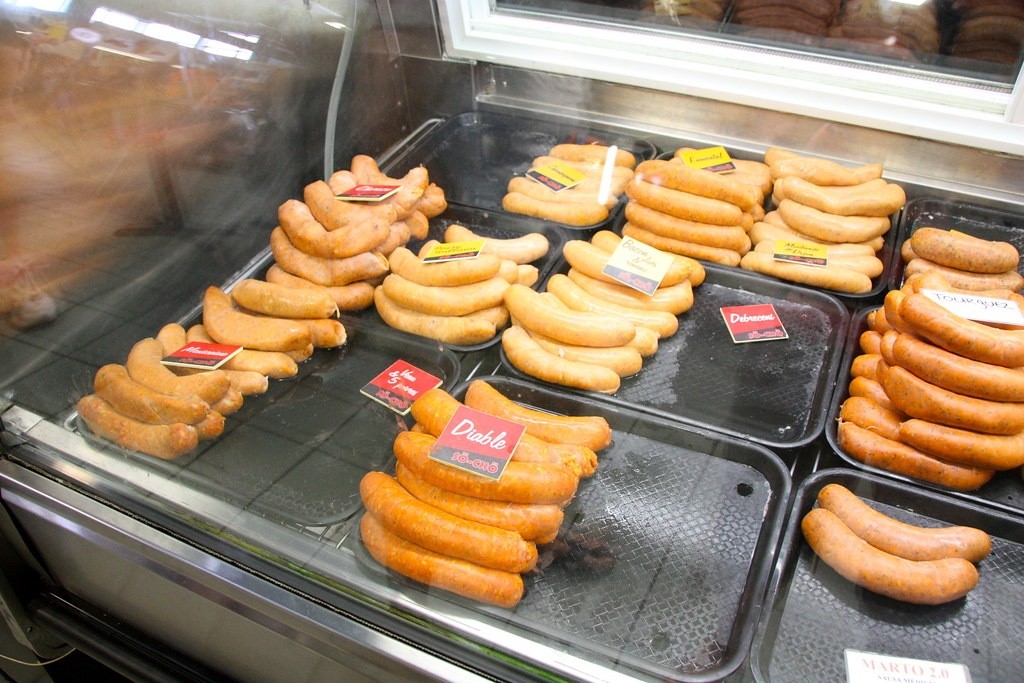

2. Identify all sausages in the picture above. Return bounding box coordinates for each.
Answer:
[77,142,1023,610]
[637,0,1022,80]
